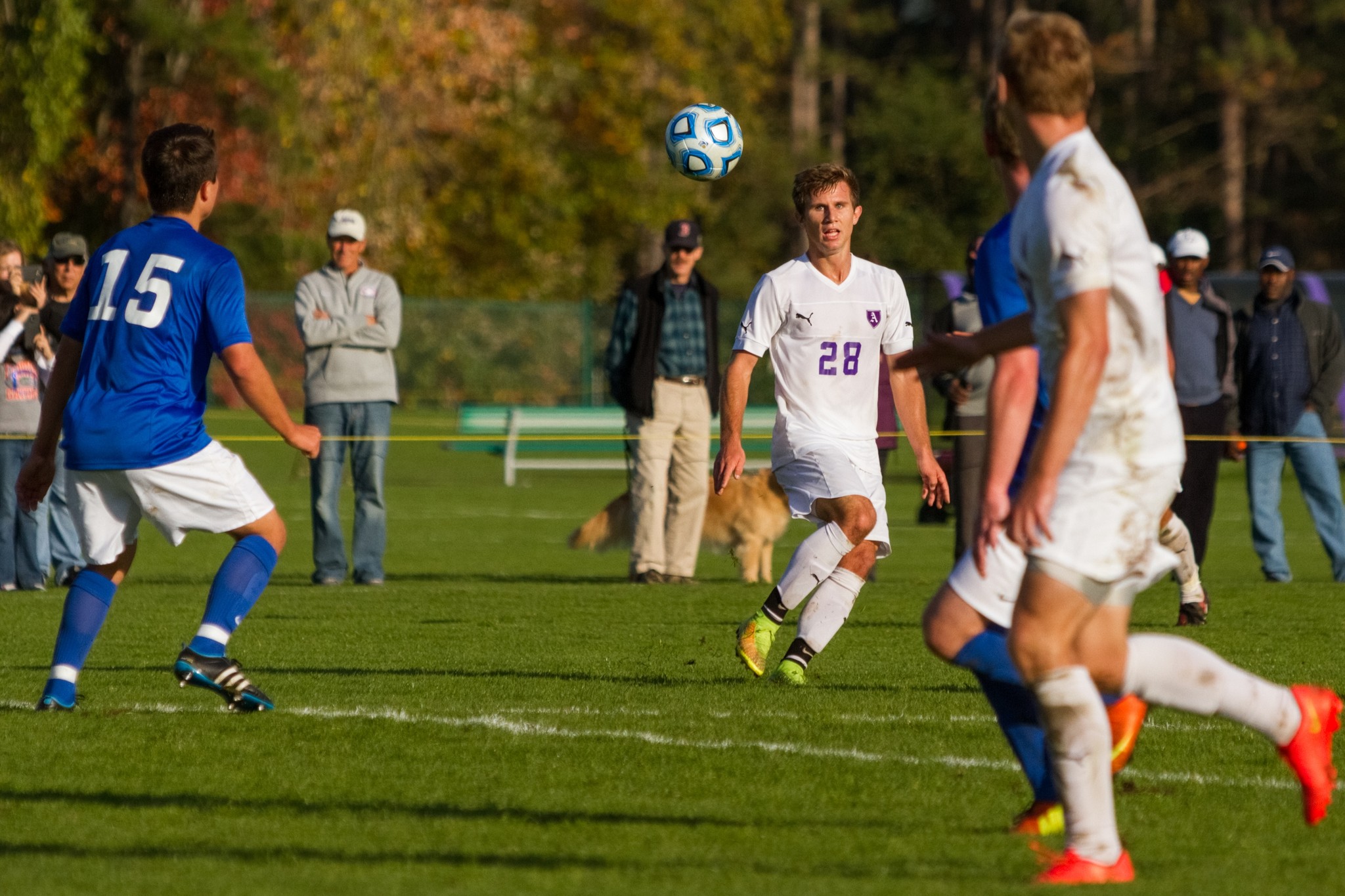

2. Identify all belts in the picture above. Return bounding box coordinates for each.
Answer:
[654,374,705,385]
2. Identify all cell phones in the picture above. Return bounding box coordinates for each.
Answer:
[24,265,44,282]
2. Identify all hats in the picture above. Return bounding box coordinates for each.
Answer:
[52,232,86,258]
[666,220,702,247]
[1260,246,1295,272]
[1167,228,1210,259]
[328,209,367,241]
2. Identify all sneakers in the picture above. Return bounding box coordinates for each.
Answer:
[771,660,808,687]
[174,642,274,712]
[35,694,85,710]
[1175,589,1208,625]
[1027,841,1136,884]
[1279,684,1343,828]
[735,609,780,678]
[1109,695,1147,775]
[1011,801,1066,836]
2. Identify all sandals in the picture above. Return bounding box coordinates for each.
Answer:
[25,583,46,591]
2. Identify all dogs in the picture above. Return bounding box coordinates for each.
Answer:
[567,465,793,586]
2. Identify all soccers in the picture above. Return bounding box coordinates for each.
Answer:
[664,102,745,181]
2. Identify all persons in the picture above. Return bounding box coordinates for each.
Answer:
[920,230,1344,630]
[292,209,403,587]
[995,6,1344,883]
[15,122,322,714]
[0,231,91,593]
[920,71,1150,839]
[603,220,723,586]
[714,163,952,686]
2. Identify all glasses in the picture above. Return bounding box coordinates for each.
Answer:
[967,257,976,267]
[53,255,85,265]
[670,246,696,254]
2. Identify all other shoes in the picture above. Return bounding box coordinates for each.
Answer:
[355,576,382,585]
[628,565,665,584]
[55,568,76,587]
[665,575,692,584]
[2,583,17,591]
[321,577,340,585]
